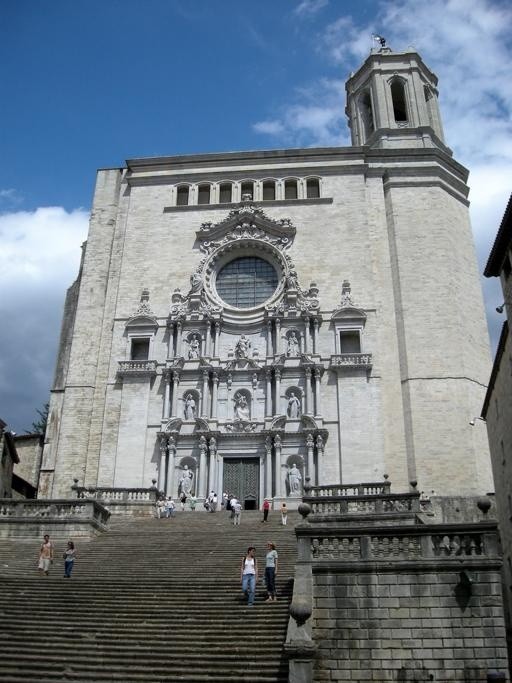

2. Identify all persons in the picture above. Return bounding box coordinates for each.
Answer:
[37,533,54,577]
[230,495,238,518]
[261,498,270,522]
[288,461,302,495]
[178,394,196,420]
[281,502,288,526]
[231,391,250,420]
[232,501,243,525]
[239,545,259,606]
[261,541,278,602]
[288,392,300,418]
[179,463,194,496]
[234,333,251,358]
[63,540,77,578]
[287,331,299,357]
[156,489,229,519]
[183,334,200,360]
